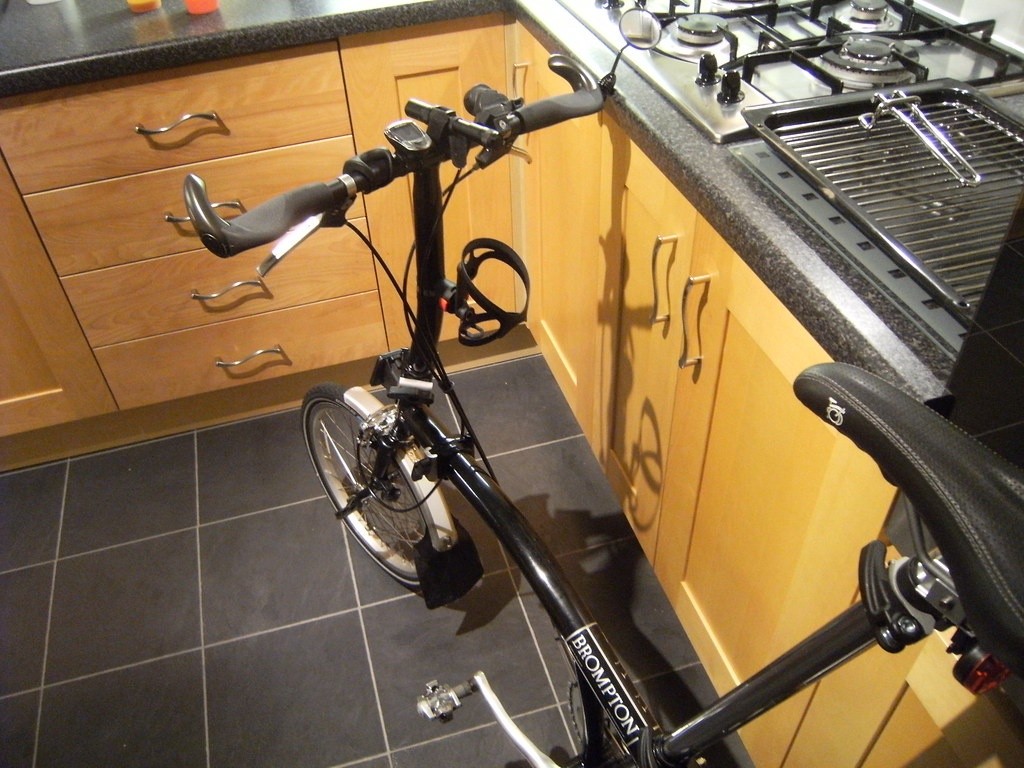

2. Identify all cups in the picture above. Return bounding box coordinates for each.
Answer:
[182,0,220,14]
[127,0,162,13]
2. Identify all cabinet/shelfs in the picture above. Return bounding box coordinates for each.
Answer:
[515,21,1024,768]
[0,14,518,472]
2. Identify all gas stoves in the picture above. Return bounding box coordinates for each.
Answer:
[555,0,1024,145]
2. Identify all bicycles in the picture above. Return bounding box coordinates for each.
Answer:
[181,53,1024,768]
